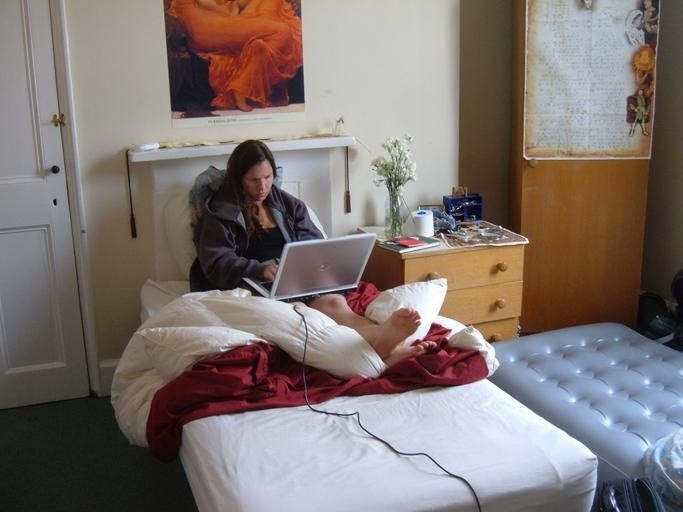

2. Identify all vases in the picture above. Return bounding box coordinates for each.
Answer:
[385,188,403,238]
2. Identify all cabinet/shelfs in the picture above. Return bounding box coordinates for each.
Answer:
[358,221,530,343]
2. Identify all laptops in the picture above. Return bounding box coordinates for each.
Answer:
[241,232,378,302]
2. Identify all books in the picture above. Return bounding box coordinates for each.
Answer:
[376,233,441,254]
[389,234,425,246]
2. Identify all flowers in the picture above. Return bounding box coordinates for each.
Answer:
[370,134,417,233]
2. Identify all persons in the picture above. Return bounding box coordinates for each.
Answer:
[189,139,437,368]
[171,0,291,111]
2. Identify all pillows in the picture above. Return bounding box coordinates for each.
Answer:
[190,165,284,240]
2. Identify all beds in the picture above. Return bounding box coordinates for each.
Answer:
[139,182,598,512]
